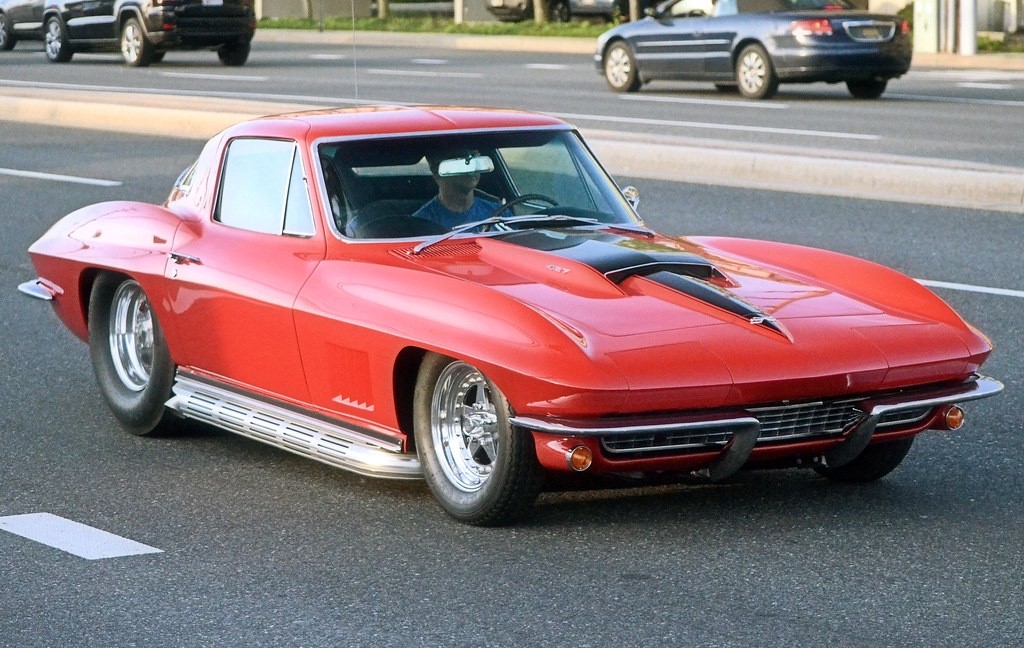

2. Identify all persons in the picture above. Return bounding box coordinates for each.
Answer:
[410,137,520,233]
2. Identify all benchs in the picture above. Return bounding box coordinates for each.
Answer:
[355,199,426,231]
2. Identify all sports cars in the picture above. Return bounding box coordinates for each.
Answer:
[18,105,1005,530]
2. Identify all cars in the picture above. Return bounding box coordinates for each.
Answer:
[591,0,912,101]
[484,0,615,20]
[0,1,51,51]
[42,0,257,71]
